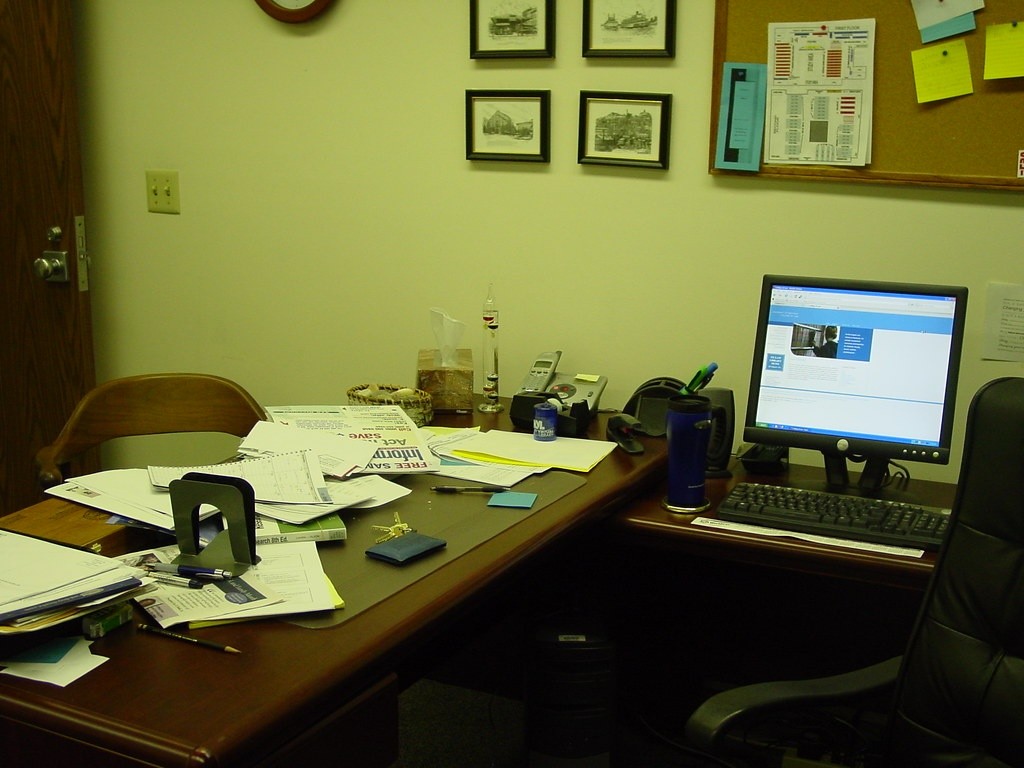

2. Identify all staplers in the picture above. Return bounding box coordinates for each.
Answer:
[606,414,645,454]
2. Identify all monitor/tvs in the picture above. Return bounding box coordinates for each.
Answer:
[743,275,969,501]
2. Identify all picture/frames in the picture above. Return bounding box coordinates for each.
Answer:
[582,0,676,58]
[470,0,555,58]
[464,89,550,163]
[577,90,672,169]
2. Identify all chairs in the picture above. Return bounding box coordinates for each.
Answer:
[36,372,267,496]
[685,377,1024,768]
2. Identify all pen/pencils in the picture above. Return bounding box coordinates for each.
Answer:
[138,623,242,655]
[144,571,203,589]
[676,362,719,395]
[146,560,233,580]
[429,484,512,495]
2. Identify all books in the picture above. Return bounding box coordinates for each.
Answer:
[791,323,823,357]
[219,508,347,543]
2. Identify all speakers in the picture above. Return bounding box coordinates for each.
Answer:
[697,387,733,480]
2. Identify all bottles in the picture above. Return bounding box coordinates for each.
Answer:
[660,394,711,513]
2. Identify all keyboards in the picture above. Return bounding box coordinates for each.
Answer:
[715,482,953,552]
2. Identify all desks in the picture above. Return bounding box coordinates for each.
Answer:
[0,397,675,768]
[608,463,954,578]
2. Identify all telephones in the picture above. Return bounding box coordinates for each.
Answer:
[515,351,609,421]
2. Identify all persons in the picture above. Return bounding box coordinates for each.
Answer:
[808,326,838,358]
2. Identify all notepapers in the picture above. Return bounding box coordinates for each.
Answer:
[486,491,537,509]
